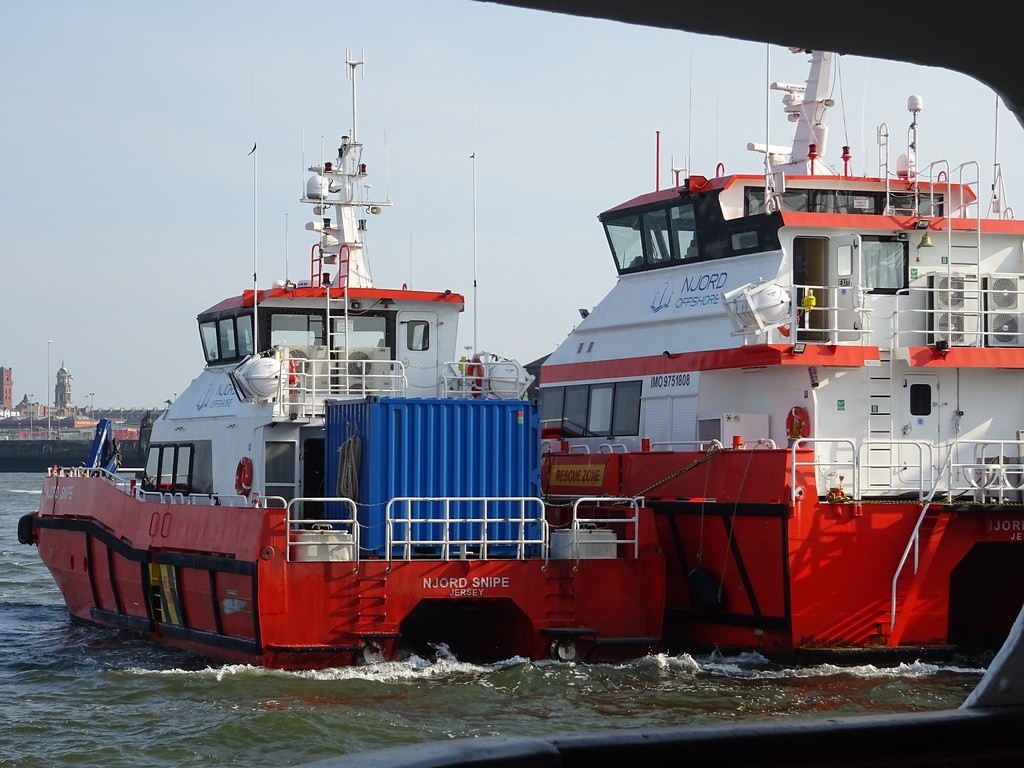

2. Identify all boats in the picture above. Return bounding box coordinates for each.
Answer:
[539,30,1024,672]
[17,42,667,674]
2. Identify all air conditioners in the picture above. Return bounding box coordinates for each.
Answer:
[980,273,1024,347]
[335,346,395,393]
[286,346,328,395]
[924,270,983,346]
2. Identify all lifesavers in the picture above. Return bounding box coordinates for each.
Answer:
[786,406,810,446]
[289,354,295,383]
[235,457,252,497]
[467,357,484,398]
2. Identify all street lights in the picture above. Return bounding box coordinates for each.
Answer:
[45,341,54,440]
[28,393,35,436]
[173,393,177,400]
[89,392,95,438]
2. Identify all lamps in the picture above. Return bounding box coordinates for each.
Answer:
[916,231,933,261]
